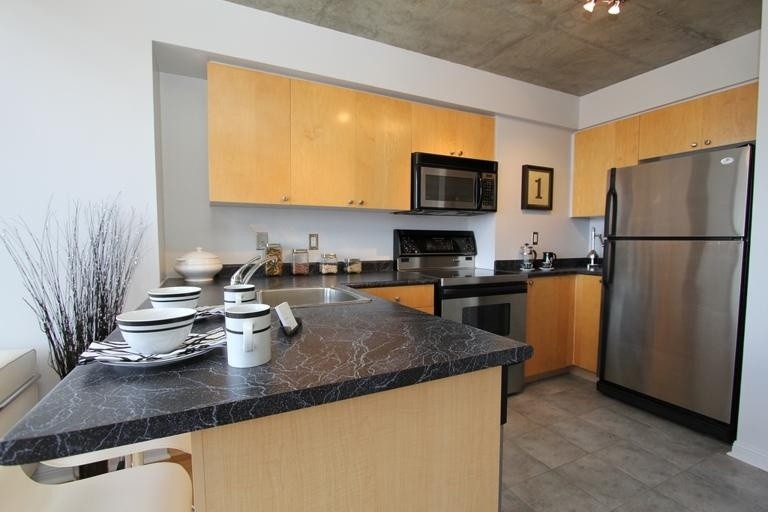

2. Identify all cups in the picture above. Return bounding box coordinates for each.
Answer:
[114,309,201,353]
[519,262,553,273]
[227,304,271,372]
[222,286,257,333]
[145,283,201,312]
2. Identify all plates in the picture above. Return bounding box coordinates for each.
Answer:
[92,333,214,370]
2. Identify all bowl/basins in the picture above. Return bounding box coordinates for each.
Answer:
[173,245,224,283]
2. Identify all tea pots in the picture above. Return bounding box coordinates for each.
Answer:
[543,251,557,271]
[518,243,536,268]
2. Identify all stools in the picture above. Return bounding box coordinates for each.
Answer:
[36,429,195,471]
[0,436,193,511]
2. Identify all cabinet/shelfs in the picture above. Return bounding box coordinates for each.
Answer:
[521,272,577,386]
[569,115,638,218]
[203,56,291,207]
[291,75,411,216]
[411,96,497,161]
[353,282,435,318]
[569,270,603,384]
[638,75,758,161]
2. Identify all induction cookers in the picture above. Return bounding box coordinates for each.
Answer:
[413,268,526,286]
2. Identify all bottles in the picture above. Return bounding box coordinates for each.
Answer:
[265,244,283,276]
[346,258,362,274]
[318,250,338,274]
[292,249,310,277]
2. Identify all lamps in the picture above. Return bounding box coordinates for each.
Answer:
[582,1,630,17]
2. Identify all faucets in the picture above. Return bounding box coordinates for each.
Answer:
[230,255,279,284]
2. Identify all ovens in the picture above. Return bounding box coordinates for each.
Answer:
[437,290,525,397]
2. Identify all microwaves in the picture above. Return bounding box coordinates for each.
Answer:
[411,152,499,211]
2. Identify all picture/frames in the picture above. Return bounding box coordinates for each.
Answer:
[520,162,554,211]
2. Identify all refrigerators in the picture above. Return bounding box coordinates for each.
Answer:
[594,144,752,444]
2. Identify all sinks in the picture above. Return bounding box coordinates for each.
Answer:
[256,288,373,309]
